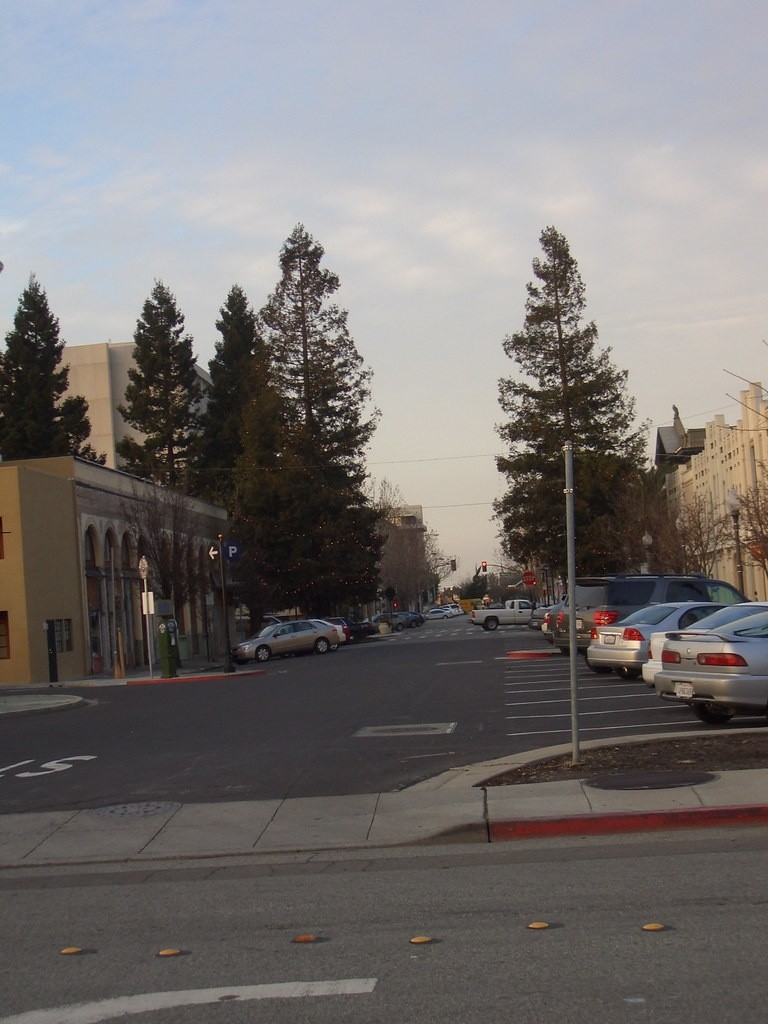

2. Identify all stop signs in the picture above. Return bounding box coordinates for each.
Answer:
[522,572,536,585]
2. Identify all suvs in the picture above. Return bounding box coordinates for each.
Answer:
[552,572,754,674]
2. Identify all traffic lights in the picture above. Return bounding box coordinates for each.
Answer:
[482,562,487,572]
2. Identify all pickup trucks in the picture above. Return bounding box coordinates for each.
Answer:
[470,599,534,631]
[339,617,380,643]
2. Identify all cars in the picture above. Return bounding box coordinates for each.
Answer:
[528,603,563,631]
[230,620,340,663]
[426,609,453,619]
[326,618,351,640]
[541,595,566,641]
[409,612,427,625]
[653,609,768,725]
[442,605,460,617]
[396,612,420,628]
[586,601,727,680]
[309,618,347,644]
[362,613,406,631]
[641,601,768,686]
